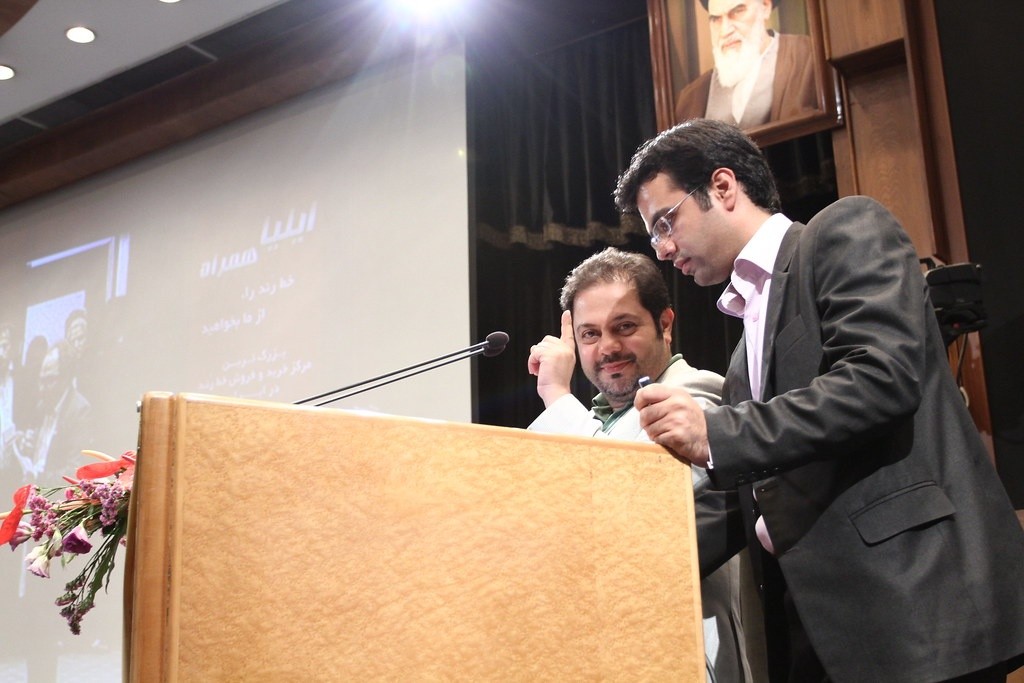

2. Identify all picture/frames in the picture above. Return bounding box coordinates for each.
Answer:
[647,1,844,155]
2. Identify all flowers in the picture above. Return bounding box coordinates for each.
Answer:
[0,448,138,635]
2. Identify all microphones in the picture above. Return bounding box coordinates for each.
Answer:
[293,332,510,406]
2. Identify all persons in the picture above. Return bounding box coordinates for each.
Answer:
[523,248,753,682]
[0,308,141,487]
[611,119,1024,682]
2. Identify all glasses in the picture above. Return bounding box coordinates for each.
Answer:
[650,185,701,251]
